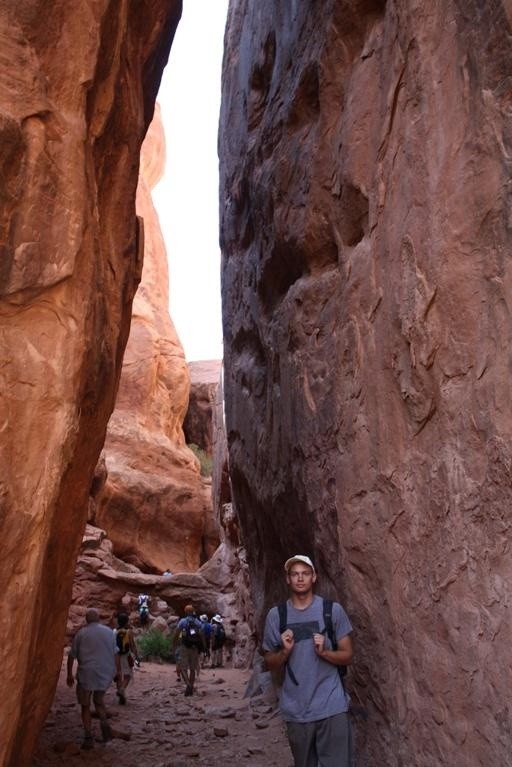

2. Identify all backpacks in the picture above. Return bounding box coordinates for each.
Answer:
[182,618,202,649]
[116,629,130,655]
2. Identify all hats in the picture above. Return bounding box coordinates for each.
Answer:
[212,615,223,623]
[284,555,317,585]
[184,605,193,613]
[199,614,208,622]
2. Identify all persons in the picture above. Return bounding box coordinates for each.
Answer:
[162,568,173,576]
[65,607,123,751]
[261,555,355,766]
[112,591,228,704]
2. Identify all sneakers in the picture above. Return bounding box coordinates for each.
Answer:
[99,717,112,740]
[81,737,94,749]
[116,690,125,705]
[185,685,192,696]
